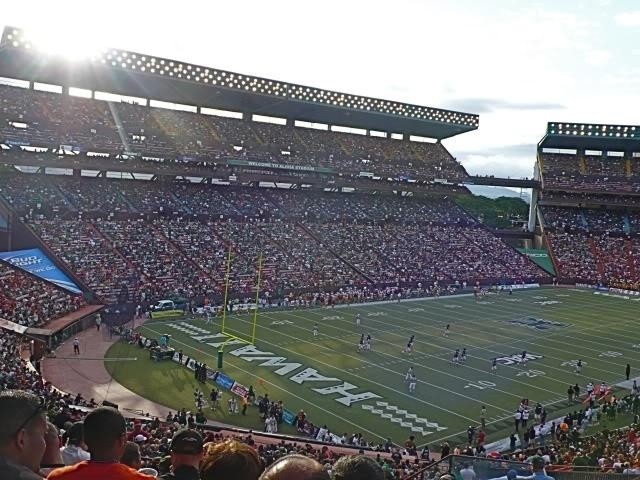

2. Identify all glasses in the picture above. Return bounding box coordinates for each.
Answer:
[15,395,46,435]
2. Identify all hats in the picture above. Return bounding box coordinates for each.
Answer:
[527,455,545,464]
[134,434,148,442]
[507,469,518,478]
[168,428,203,455]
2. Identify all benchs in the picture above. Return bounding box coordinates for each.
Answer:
[0,279,82,325]
